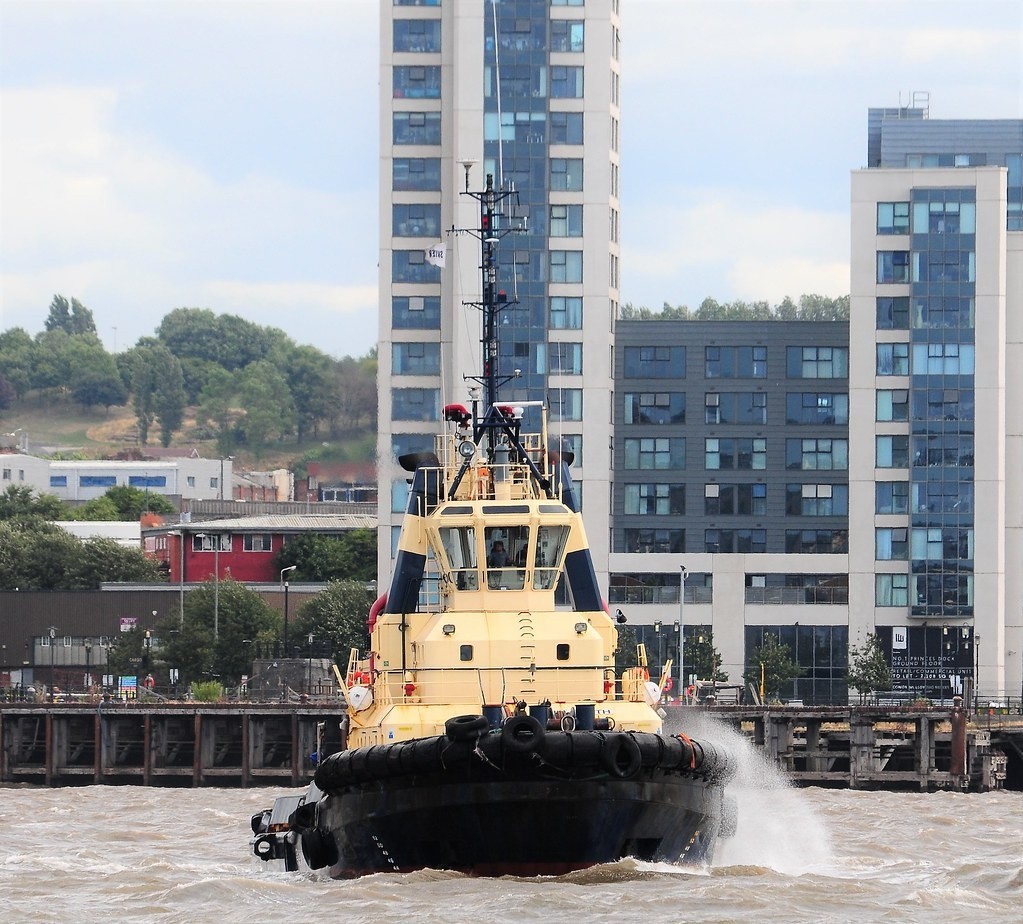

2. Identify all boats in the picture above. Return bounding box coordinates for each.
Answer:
[243,0,744,879]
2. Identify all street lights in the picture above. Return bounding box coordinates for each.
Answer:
[279,566,296,592]
[145,630,152,689]
[194,533,220,648]
[654,619,680,664]
[283,582,291,658]
[304,633,317,695]
[48,625,58,702]
[972,633,980,696]
[85,641,93,694]
[942,621,971,697]
[168,531,186,636]
[190,498,203,513]
[104,640,112,692]
[241,639,252,676]
[683,627,705,685]
[677,564,688,701]
[151,608,159,640]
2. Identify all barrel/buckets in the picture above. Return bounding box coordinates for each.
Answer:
[529,706,550,730]
[482,705,502,734]
[575,705,595,730]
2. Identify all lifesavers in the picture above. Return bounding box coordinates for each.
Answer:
[687,686,698,698]
[443,713,490,741]
[603,733,642,780]
[503,714,546,757]
[662,678,673,692]
[301,827,328,872]
[144,676,155,688]
[349,671,370,688]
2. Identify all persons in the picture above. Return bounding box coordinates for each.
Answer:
[536,549,542,566]
[516,544,528,566]
[487,542,515,567]
[309,752,324,765]
[53,687,60,694]
